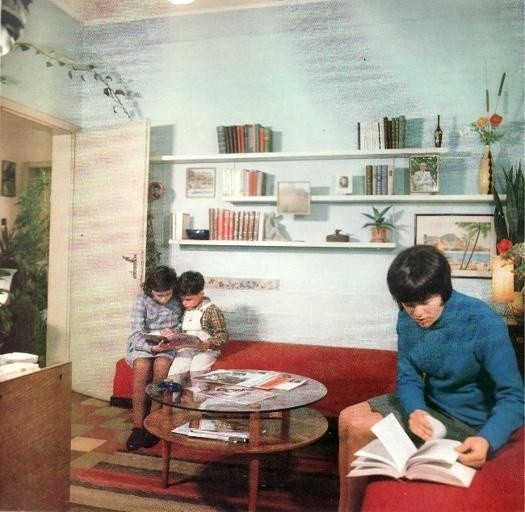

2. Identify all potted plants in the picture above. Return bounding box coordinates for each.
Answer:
[362,205,395,243]
[492,162,525,323]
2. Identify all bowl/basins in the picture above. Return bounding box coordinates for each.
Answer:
[185,229,210,239]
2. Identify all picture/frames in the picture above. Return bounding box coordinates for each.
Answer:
[414,213,499,279]
[409,154,439,192]
[277,181,311,216]
[186,168,216,199]
[335,174,352,193]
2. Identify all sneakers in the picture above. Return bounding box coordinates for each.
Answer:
[126,428,160,450]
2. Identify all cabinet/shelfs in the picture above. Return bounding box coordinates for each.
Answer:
[149,148,507,249]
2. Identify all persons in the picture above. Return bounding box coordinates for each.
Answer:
[412,163,436,191]
[166,271,229,403]
[124,264,183,452]
[325,244,525,512]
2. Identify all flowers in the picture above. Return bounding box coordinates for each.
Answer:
[460,72,507,146]
[496,238,525,267]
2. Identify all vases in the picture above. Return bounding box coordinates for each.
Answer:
[478,144,495,195]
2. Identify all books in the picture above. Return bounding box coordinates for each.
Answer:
[138,330,208,351]
[163,114,406,241]
[345,412,477,490]
[170,368,310,443]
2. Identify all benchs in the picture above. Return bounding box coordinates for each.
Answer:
[110,341,524,512]
